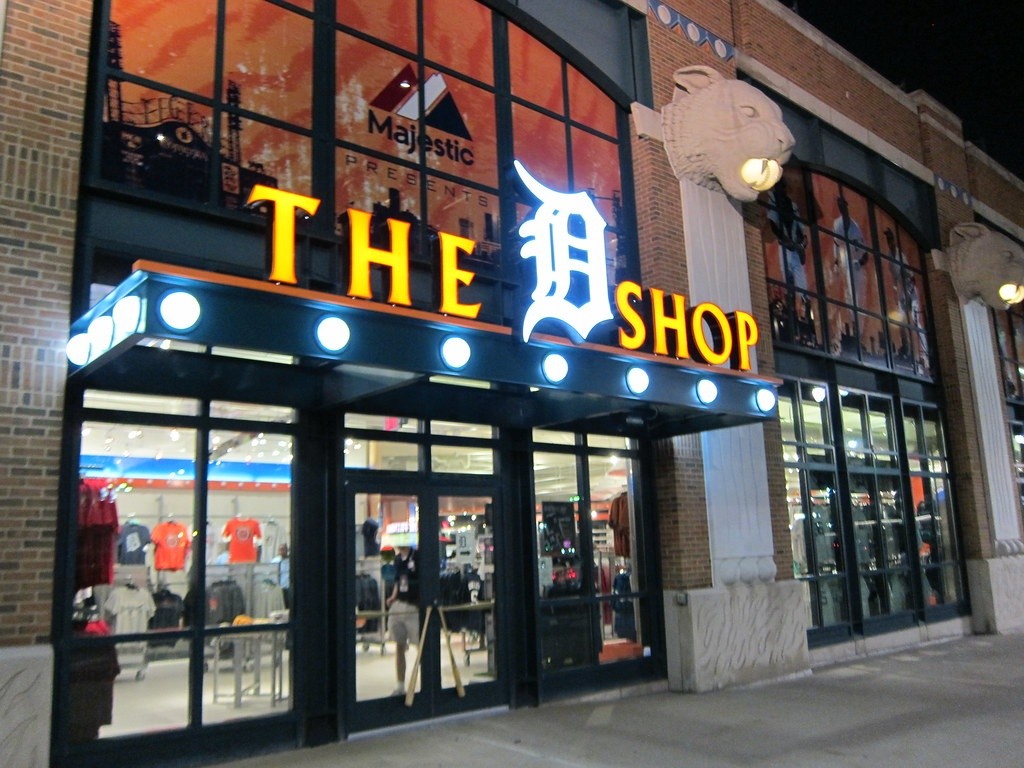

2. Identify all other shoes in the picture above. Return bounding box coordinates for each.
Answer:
[391,690,406,697]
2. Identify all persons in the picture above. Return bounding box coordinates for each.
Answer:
[278,544,420,695]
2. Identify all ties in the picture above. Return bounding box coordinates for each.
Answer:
[278,558,283,584]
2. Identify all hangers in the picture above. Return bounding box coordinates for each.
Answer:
[157,583,171,596]
[211,572,235,586]
[167,513,177,526]
[125,576,136,590]
[266,514,277,526]
[206,515,211,526]
[127,513,139,528]
[235,513,251,521]
[262,570,276,587]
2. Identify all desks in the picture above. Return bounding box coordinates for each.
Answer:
[213,618,289,708]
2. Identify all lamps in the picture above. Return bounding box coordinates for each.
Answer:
[87,316,114,352]
[66,332,91,367]
[695,376,718,404]
[112,294,142,335]
[155,288,204,335]
[625,365,650,396]
[439,335,472,371]
[542,352,569,385]
[755,387,776,414]
[313,313,352,355]
